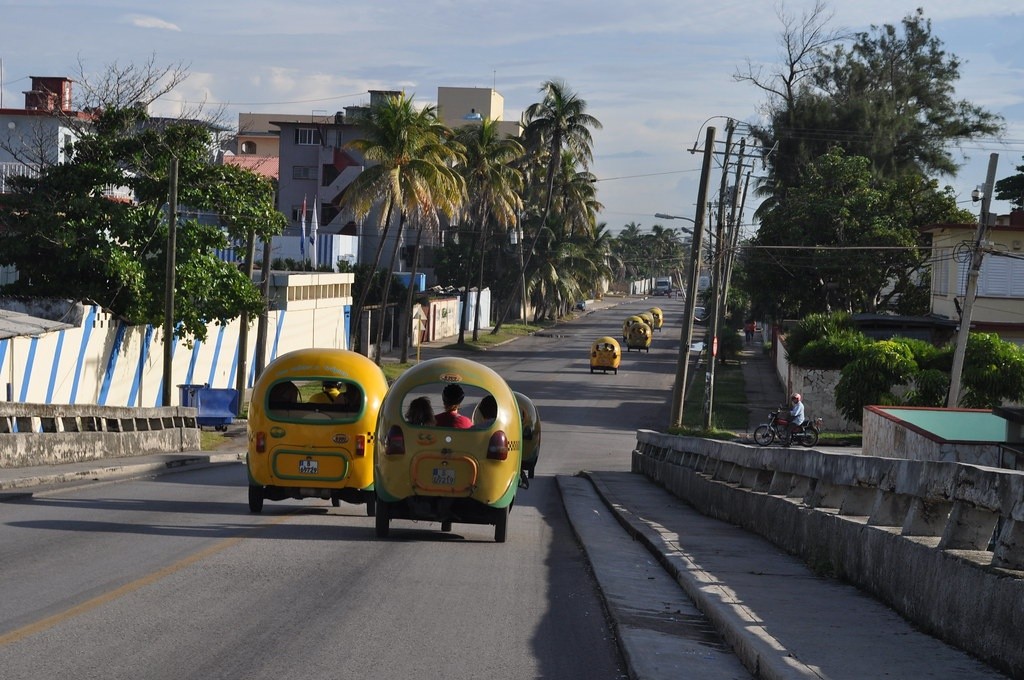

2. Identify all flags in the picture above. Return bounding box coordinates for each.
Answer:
[300,197,318,270]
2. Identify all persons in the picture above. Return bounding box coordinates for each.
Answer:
[405,397,437,427]
[745,323,751,341]
[309,380,343,404]
[434,384,473,429]
[334,382,362,404]
[751,323,754,340]
[783,393,804,447]
[596,343,614,351]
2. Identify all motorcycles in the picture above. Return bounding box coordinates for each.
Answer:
[247,349,389,516]
[590,336,621,375]
[623,307,662,342]
[627,323,652,352]
[754,403,823,448]
[373,357,541,543]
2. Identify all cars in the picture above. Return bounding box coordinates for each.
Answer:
[693,308,705,323]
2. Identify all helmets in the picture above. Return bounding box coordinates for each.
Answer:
[790,392,801,402]
[322,380,342,389]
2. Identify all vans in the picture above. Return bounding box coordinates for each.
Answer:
[698,277,710,291]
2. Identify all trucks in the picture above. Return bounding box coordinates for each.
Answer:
[656,276,672,295]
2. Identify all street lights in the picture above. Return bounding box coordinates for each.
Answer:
[655,213,731,378]
[680,226,732,364]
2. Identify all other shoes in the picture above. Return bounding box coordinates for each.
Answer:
[781,443,793,447]
